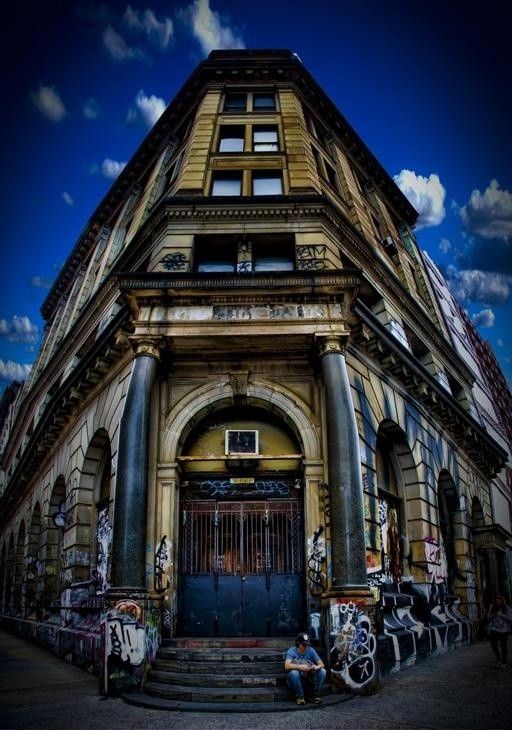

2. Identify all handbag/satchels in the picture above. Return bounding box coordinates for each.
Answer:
[292,658,314,676]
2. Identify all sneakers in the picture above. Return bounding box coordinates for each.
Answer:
[309,695,323,704]
[296,694,305,704]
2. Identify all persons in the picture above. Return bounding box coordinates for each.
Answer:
[487,597,512,669]
[284,633,327,706]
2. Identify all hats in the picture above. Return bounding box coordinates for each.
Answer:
[298,632,310,644]
